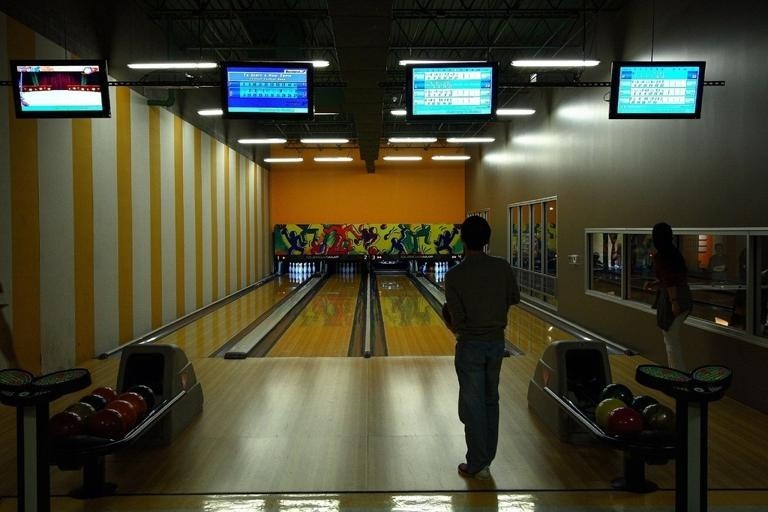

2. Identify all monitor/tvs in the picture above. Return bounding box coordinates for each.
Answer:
[10,58,111,118]
[220,60,315,122]
[406,63,497,123]
[608,60,706,119]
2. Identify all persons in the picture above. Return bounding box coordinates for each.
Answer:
[708,243,728,282]
[644,222,694,369]
[442,215,522,479]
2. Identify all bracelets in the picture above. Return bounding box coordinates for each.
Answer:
[669,297,676,302]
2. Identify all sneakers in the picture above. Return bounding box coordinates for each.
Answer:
[458,464,490,481]
